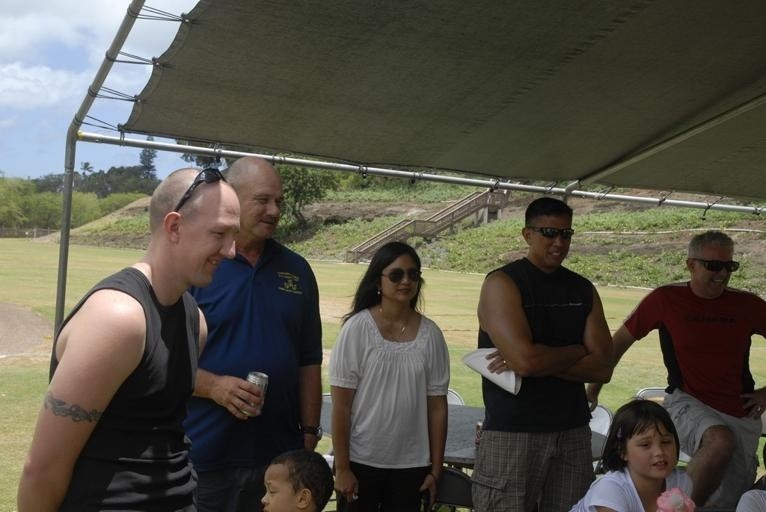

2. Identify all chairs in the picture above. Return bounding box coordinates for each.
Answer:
[636,386,692,463]
[447,389,465,407]
[588,403,613,437]
[320,393,337,501]
[433,465,473,512]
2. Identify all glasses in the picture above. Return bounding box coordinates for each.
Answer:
[690,258,740,273]
[527,226,575,240]
[381,270,422,284]
[173,167,228,212]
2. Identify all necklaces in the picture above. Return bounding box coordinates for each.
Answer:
[379,306,409,341]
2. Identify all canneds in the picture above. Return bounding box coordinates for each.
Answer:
[475,420,483,447]
[243,371,269,418]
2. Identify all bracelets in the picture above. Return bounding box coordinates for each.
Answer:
[430,472,437,482]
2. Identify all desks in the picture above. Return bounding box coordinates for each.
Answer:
[314,401,608,512]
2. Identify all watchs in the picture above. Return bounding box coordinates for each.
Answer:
[300,426,323,440]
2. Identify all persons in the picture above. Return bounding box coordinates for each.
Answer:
[187,156,323,512]
[331,241,451,512]
[262,450,335,512]
[18,168,242,511]
[585,231,765,511]
[569,400,695,511]
[737,489,766,512]
[472,197,616,511]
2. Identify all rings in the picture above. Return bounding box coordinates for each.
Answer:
[757,406,761,411]
[503,360,507,364]
